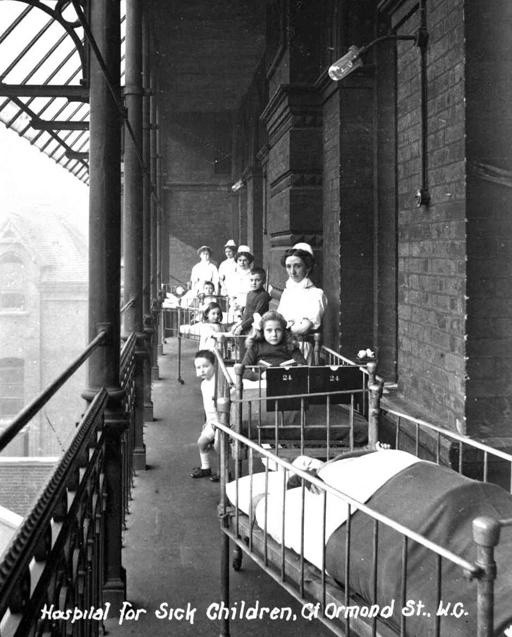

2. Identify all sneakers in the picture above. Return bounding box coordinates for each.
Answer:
[210,471,231,481]
[190,468,211,478]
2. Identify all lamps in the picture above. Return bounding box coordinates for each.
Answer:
[230,171,268,193]
[325,28,419,84]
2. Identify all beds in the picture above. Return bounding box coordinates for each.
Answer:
[157,282,512,636]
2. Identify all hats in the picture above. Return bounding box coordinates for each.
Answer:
[238,246,252,255]
[225,240,236,247]
[292,242,314,257]
[197,245,212,256]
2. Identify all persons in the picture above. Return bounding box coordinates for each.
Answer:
[271,241,328,361]
[284,438,391,495]
[177,232,273,360]
[241,311,308,382]
[189,348,235,480]
